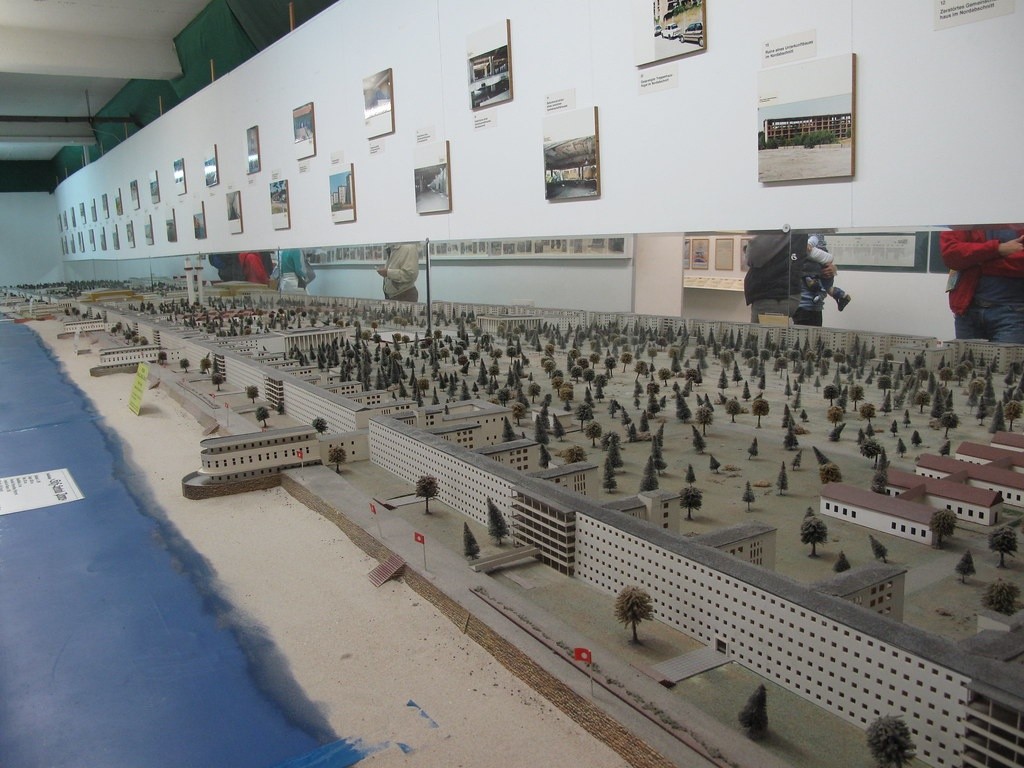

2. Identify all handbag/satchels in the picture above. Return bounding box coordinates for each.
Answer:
[296,259,315,288]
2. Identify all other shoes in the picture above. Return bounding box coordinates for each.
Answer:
[837,293,851,311]
[813,288,827,303]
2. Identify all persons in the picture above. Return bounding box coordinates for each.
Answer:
[743,230,850,328]
[377,245,421,303]
[937,223,1024,343]
[206,250,309,294]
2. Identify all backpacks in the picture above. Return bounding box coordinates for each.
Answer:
[209,253,250,282]
